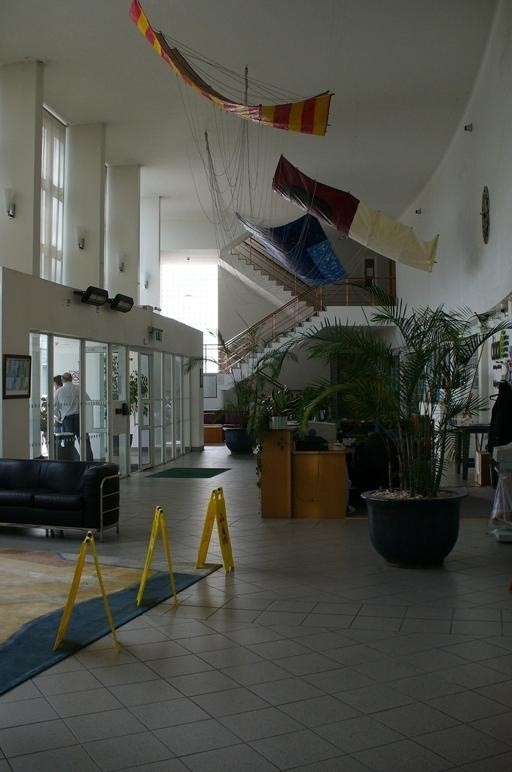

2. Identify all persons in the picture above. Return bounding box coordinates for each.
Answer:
[54,373,93,461]
[54,375,63,433]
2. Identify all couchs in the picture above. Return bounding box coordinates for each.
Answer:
[0,457,121,542]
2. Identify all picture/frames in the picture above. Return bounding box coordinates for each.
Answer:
[4,354,32,399]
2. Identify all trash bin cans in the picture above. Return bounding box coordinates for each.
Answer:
[53,432,75,461]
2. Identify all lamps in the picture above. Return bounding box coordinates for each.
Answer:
[74,286,134,312]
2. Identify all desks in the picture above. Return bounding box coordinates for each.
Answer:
[446,424,499,480]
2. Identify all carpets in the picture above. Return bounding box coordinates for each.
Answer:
[1,546,223,694]
[146,467,232,479]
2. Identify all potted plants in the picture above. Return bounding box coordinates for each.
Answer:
[304,288,512,569]
[183,313,301,458]
[104,354,149,456]
